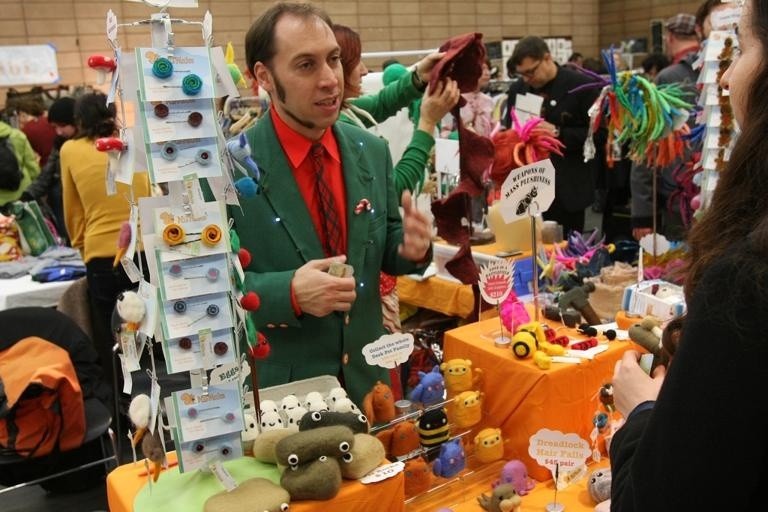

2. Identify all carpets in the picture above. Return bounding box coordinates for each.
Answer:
[309,142,345,255]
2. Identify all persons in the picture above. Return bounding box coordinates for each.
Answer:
[18,96,78,247]
[217,3,434,412]
[59,92,153,434]
[508,36,610,239]
[480,59,503,95]
[569,13,702,259]
[608,1,768,511]
[1,84,67,210]
[332,25,461,207]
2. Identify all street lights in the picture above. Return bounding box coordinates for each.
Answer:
[0,337,87,461]
[13,201,56,256]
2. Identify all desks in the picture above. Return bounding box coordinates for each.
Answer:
[1,173,686,511]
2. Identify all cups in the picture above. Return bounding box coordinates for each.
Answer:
[516,59,541,78]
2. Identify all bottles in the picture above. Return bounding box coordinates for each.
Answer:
[9,98,43,118]
[662,13,698,34]
[48,97,75,126]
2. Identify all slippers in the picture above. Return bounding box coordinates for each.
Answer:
[0,134,27,191]
